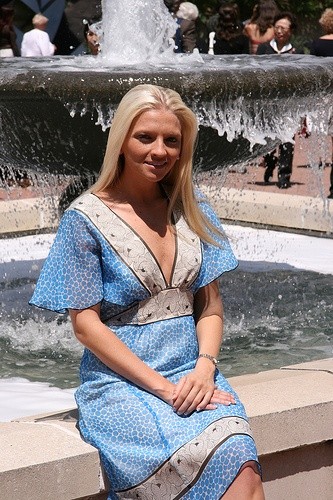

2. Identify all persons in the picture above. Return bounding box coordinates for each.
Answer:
[255,11,303,188]
[29,84,267,500]
[0,0,101,185]
[168,0,279,54]
[311,7,333,169]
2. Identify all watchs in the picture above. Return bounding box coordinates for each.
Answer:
[198,354,217,367]
